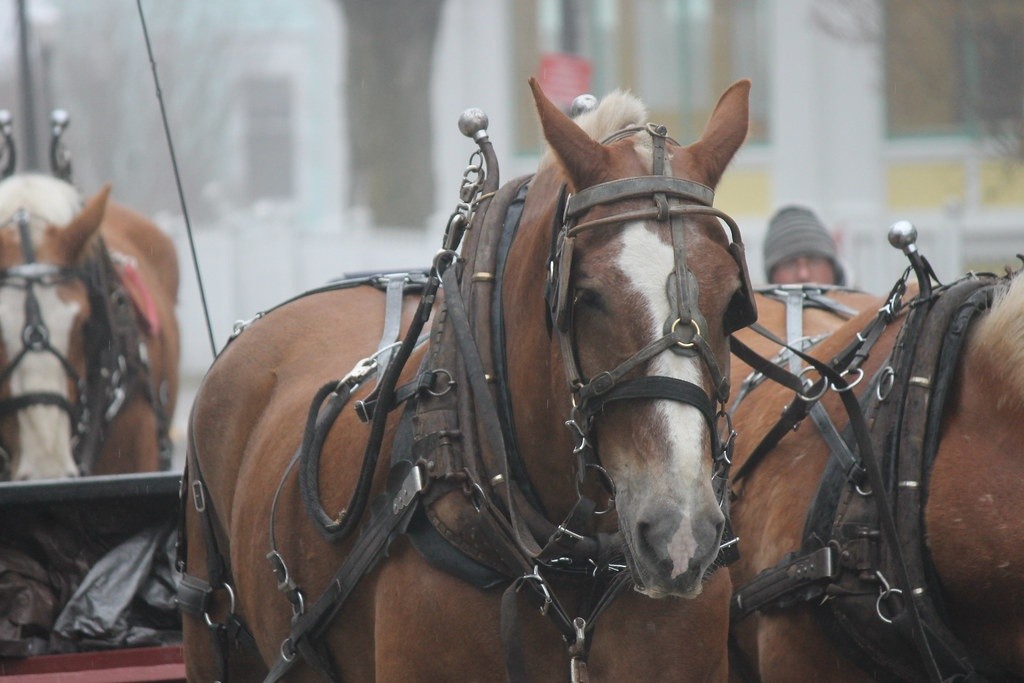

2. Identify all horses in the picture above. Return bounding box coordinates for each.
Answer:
[0,169,179,481]
[179,76,752,683]
[726,255,1024,681]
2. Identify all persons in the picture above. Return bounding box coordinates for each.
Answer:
[765,207,848,286]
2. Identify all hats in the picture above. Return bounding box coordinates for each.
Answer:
[765,205,843,284]
